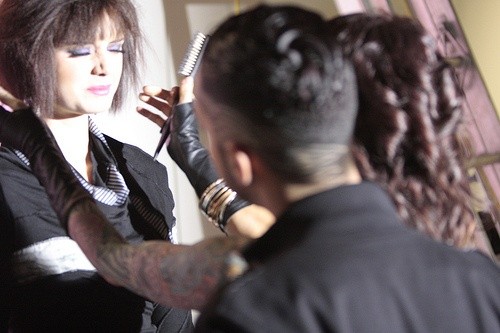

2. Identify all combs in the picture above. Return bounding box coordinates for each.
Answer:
[145,27,212,161]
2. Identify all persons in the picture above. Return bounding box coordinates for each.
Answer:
[193,2,500,333]
[0,12,478,312]
[1,0,201,333]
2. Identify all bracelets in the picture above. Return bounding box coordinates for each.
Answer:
[195,175,238,228]
